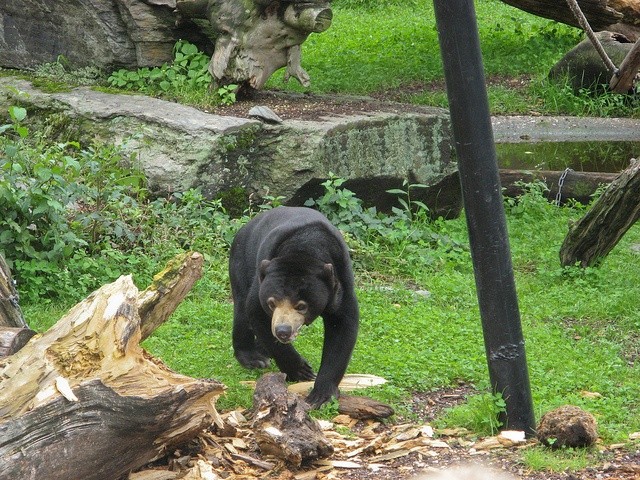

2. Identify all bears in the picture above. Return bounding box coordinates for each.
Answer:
[228,206,359,411]
